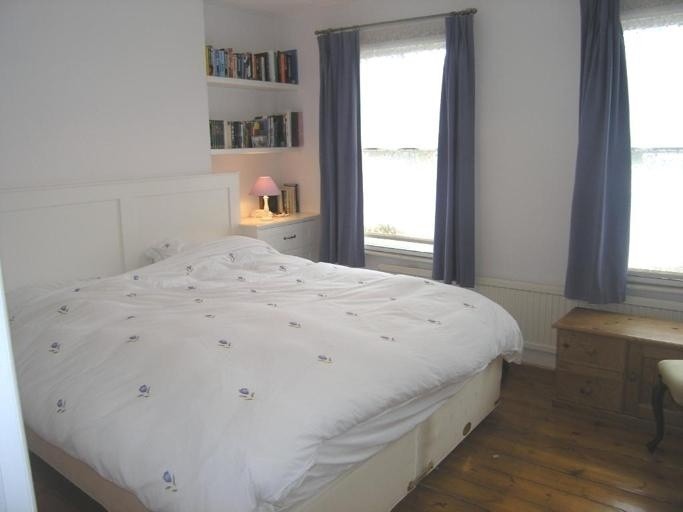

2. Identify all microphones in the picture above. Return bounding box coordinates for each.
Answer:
[645,359,682,453]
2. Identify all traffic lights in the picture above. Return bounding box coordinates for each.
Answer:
[144,231,205,265]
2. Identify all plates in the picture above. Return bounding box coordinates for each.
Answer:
[207,74,300,156]
[548,305,682,436]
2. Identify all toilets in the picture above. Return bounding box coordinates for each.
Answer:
[250,209,273,217]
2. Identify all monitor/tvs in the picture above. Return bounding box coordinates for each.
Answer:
[207,111,298,149]
[259,182,298,215]
[205,44,297,85]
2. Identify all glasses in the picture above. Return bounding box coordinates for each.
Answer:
[247,175,280,221]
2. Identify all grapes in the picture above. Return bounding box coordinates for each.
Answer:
[240,209,320,263]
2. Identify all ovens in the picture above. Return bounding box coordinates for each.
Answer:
[1,170,506,511]
